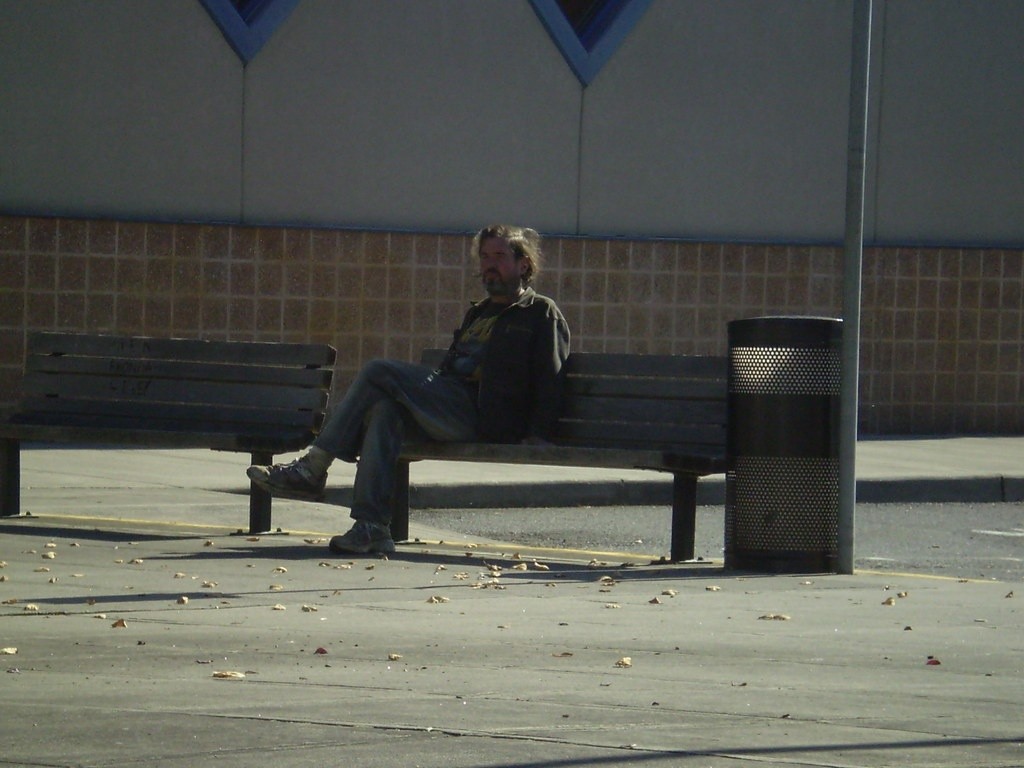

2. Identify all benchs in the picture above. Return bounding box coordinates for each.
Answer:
[0,330,337,536]
[357,349,729,564]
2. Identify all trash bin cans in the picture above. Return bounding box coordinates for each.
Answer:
[724,315,844,574]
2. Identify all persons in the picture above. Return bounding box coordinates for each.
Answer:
[246,224,571,559]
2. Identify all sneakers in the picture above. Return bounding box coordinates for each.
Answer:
[329,521,395,554]
[246,457,328,502]
